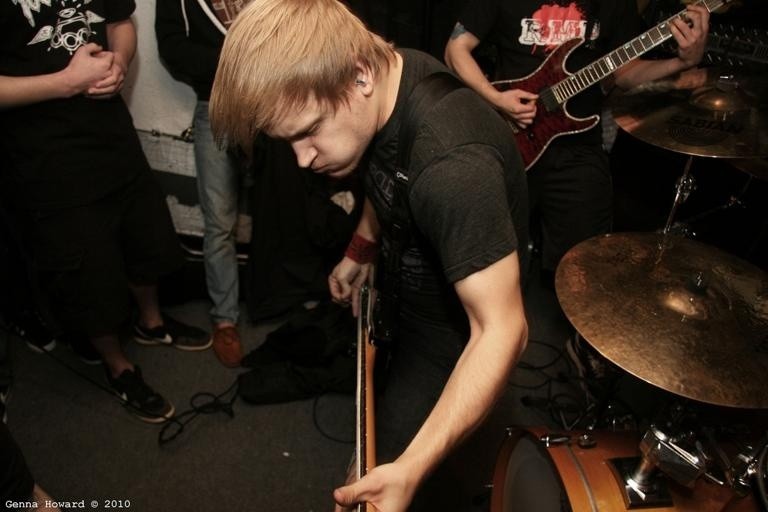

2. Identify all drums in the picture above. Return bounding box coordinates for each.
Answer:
[488,425,759,511]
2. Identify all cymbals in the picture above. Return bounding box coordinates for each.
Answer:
[612,70,767,159]
[555,230,768,409]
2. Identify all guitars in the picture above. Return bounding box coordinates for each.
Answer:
[488,0,726,171]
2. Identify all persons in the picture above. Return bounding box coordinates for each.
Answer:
[1,0,768,512]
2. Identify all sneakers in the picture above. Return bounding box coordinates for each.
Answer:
[106,364,175,422]
[3,306,53,352]
[132,314,213,351]
[213,324,245,369]
[0,374,15,427]
[59,321,103,365]
